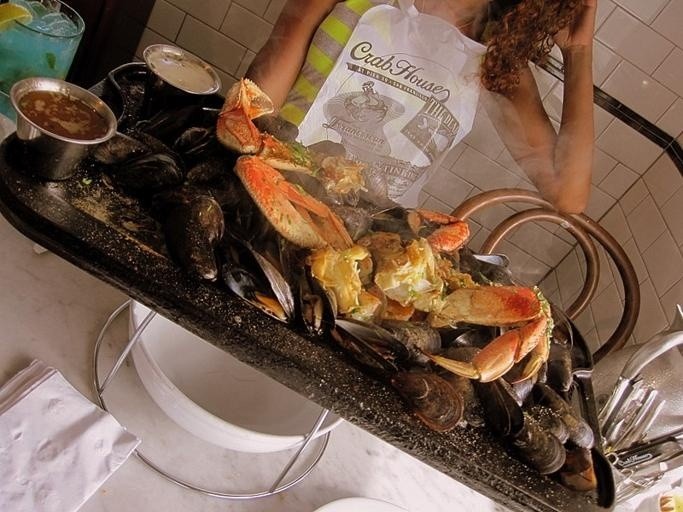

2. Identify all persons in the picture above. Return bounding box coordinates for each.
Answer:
[243,1,595,216]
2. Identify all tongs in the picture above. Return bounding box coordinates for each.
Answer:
[608,428,683,480]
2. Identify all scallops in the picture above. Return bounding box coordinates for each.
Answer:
[92,76,599,495]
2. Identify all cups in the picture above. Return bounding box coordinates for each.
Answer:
[0,0,87,123]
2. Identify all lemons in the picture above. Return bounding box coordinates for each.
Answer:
[0,3,32,31]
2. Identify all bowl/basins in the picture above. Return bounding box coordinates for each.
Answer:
[142,42,222,116]
[128,296,344,455]
[9,76,118,181]
